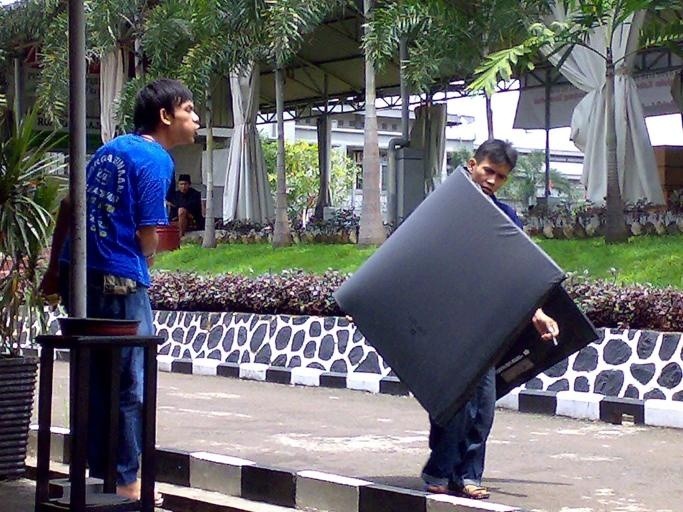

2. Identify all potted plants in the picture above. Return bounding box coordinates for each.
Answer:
[1,95,62,483]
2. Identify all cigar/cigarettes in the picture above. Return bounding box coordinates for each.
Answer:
[550,333,559,346]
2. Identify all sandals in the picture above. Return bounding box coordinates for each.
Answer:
[464,485,492,500]
[426,484,449,494]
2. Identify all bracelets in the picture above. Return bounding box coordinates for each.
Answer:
[145,252,155,260]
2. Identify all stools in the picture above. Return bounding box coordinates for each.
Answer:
[30,333,167,512]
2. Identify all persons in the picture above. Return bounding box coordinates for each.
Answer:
[165,173,203,237]
[56,77,202,506]
[418,138,563,504]
